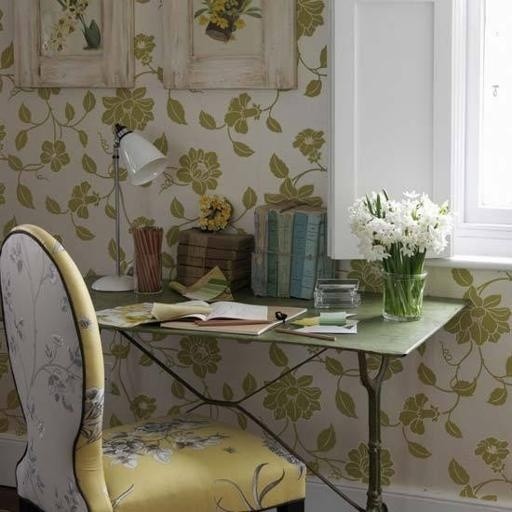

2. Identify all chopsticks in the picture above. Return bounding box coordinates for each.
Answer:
[195,320,273,325]
[132,225,164,293]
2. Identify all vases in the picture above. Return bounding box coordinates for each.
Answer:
[204,13,238,44]
[372,265,431,326]
[82,19,106,51]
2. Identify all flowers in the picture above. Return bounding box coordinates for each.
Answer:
[193,0,266,34]
[40,1,95,54]
[195,192,236,233]
[341,183,454,319]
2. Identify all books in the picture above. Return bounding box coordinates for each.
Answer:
[250,203,337,301]
[146,299,272,325]
[175,229,255,292]
[160,306,308,336]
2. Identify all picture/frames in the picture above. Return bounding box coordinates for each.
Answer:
[11,0,139,90]
[159,0,302,92]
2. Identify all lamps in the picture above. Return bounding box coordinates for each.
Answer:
[85,116,173,294]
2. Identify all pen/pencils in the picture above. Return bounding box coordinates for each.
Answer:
[133,226,163,293]
[194,319,272,326]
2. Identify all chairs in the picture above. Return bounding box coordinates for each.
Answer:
[1,216,310,511]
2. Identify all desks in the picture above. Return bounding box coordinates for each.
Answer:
[73,271,471,512]
